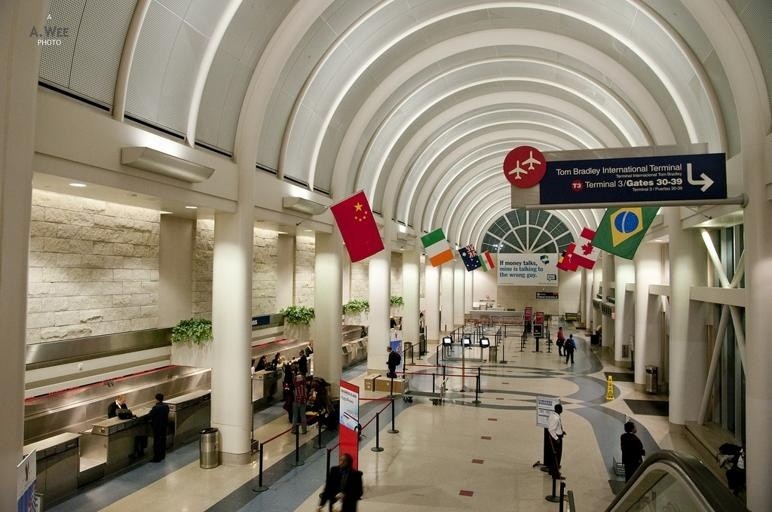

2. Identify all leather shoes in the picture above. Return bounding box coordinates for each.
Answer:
[550,471,567,480]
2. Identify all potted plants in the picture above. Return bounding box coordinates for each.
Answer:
[389,296,403,318]
[280,306,314,342]
[171,317,211,367]
[344,300,369,327]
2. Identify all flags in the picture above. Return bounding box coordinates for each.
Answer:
[457,244,481,271]
[329,190,386,263]
[591,206,661,260]
[477,252,495,273]
[420,227,454,267]
[556,227,600,273]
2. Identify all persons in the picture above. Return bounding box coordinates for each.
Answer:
[725,440,746,497]
[361,327,367,337]
[548,403,567,480]
[132,392,169,463]
[386,346,396,373]
[108,394,128,419]
[251,342,313,435]
[391,318,396,328]
[557,327,577,364]
[318,453,363,512]
[620,422,645,485]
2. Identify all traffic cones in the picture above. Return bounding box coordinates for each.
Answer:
[603,374,616,402]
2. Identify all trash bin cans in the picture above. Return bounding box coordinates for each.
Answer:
[645,365,659,394]
[199,428,219,469]
[404,342,412,365]
